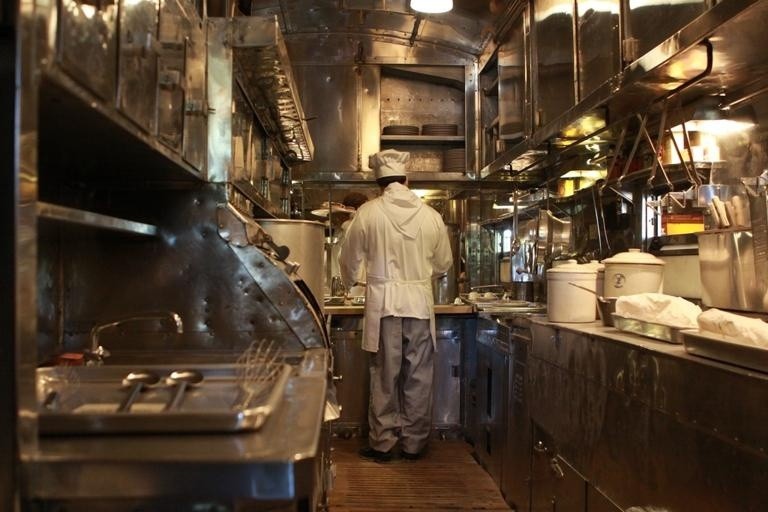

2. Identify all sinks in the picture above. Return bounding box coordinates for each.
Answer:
[495,300,545,309]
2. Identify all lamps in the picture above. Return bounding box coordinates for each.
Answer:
[492,198,530,210]
[408,0,454,15]
[557,159,608,178]
[664,95,760,136]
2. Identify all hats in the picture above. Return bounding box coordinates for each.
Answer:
[368,148,410,180]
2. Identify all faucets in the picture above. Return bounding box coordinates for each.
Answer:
[517,266,545,284]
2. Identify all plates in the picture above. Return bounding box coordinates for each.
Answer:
[442,148,466,172]
[384,124,457,136]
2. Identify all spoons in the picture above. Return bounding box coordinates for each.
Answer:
[117,367,204,412]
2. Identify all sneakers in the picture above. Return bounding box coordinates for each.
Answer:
[400,442,429,460]
[359,446,392,461]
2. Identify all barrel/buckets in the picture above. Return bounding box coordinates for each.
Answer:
[545,259,599,323]
[604,248,667,299]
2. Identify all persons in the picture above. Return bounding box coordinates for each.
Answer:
[337,148,455,462]
[332,188,374,291]
